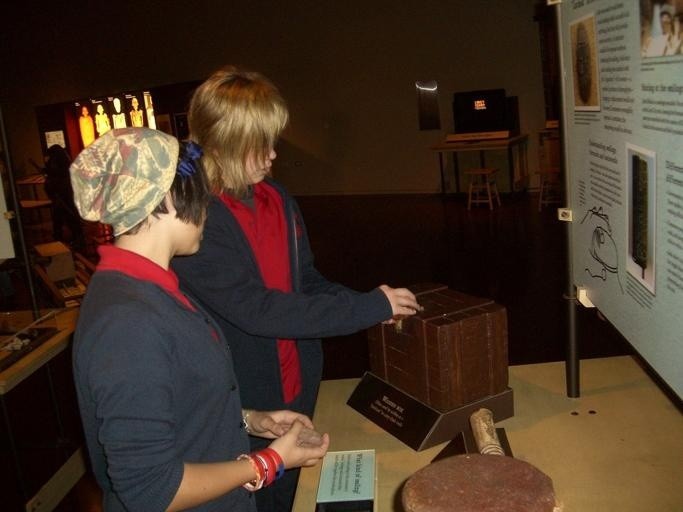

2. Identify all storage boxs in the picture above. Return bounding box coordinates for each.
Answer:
[368,282,508,414]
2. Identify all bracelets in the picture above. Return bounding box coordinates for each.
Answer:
[236,448,285,492]
[242,409,249,433]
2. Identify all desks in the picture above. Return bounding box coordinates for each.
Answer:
[429,134,528,204]
[290,356,682,512]
[0,308,79,500]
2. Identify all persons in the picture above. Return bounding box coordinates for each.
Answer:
[643,11,683,57]
[170,64,425,512]
[68,128,330,512]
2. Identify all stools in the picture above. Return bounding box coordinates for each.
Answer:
[465,167,502,210]
[535,168,561,214]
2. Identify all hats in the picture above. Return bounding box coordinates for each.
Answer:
[69,127,180,237]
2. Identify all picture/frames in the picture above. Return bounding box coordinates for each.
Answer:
[568,12,601,113]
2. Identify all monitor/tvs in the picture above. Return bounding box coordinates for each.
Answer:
[447,88,520,141]
